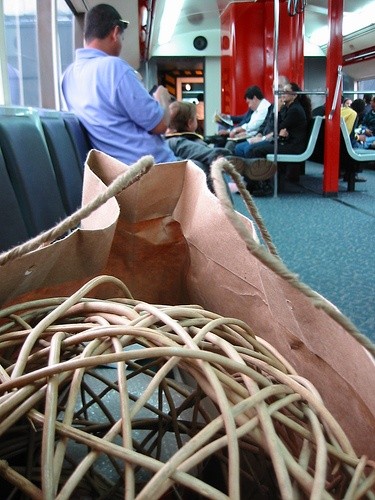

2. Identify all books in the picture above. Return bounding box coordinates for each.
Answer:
[212,110,234,127]
[227,130,256,138]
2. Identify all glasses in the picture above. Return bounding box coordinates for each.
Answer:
[115,20,130,29]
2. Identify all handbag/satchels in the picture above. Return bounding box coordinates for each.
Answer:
[1,149,375,500]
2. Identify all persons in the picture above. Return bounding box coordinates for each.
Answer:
[57,3,183,165]
[162,75,305,195]
[316,93,375,147]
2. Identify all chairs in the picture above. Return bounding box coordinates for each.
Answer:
[0,104,375,254]
